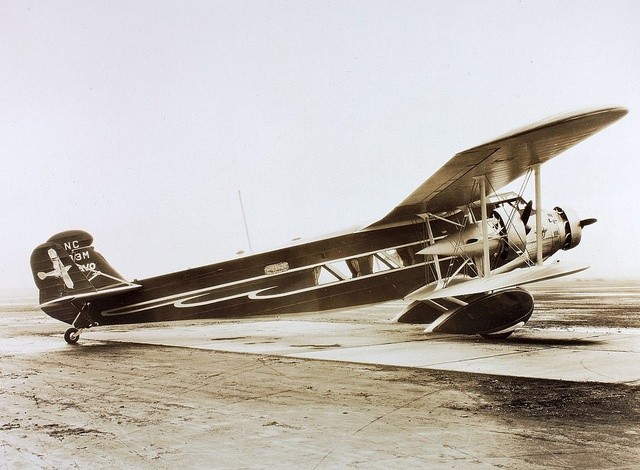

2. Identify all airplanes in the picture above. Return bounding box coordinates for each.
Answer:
[31,108,629,343]
